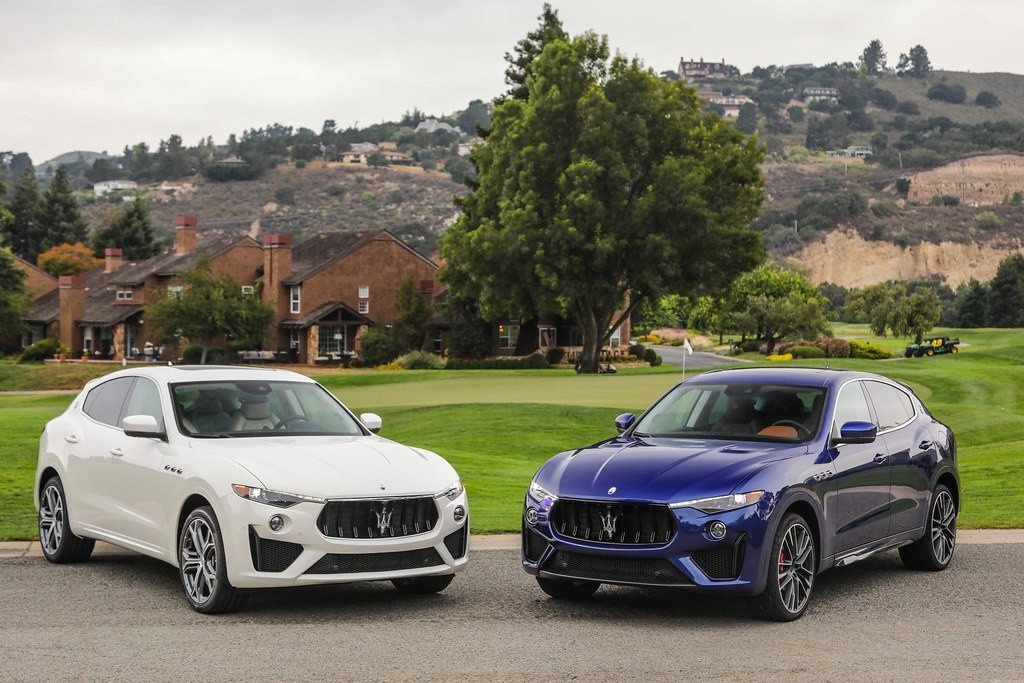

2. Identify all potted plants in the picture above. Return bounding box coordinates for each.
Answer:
[56,344,71,360]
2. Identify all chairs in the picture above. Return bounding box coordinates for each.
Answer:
[714,395,763,435]
[131,347,145,360]
[192,394,228,433]
[228,394,287,430]
[798,393,825,439]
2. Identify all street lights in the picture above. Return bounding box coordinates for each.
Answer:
[127,320,143,358]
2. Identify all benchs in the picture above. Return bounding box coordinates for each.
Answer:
[313,352,341,365]
[238,351,298,364]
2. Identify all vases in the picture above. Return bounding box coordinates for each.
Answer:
[82,356,87,362]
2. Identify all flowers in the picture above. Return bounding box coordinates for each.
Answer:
[38,242,105,276]
[83,349,88,357]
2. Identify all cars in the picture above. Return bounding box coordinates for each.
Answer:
[33,361,472,613]
[520,367,963,621]
